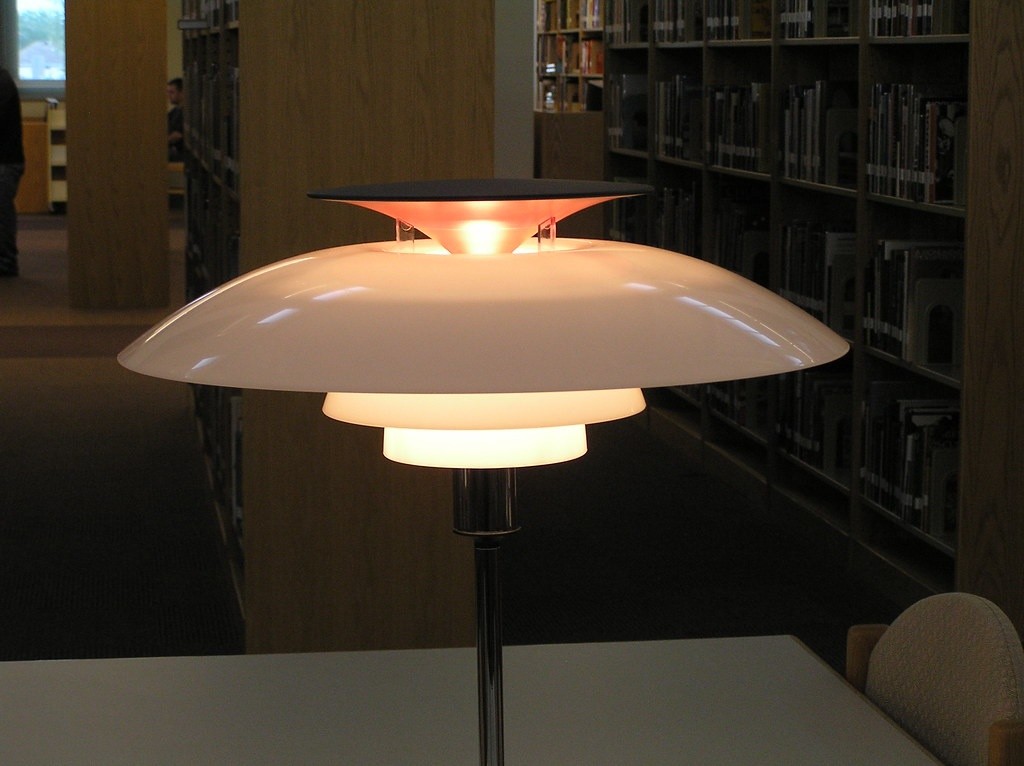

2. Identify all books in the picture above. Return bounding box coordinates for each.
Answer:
[524,0,975,548]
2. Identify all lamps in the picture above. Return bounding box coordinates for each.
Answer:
[118,177,851,766]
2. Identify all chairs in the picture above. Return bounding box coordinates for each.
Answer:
[844,591,1024,766]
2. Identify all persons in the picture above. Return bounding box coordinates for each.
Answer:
[166,77,184,161]
[0,67,26,279]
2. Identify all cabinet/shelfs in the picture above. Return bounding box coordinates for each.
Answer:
[538,1,1024,602]
[182,0,497,655]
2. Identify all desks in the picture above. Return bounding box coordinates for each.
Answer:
[0,632,942,766]
